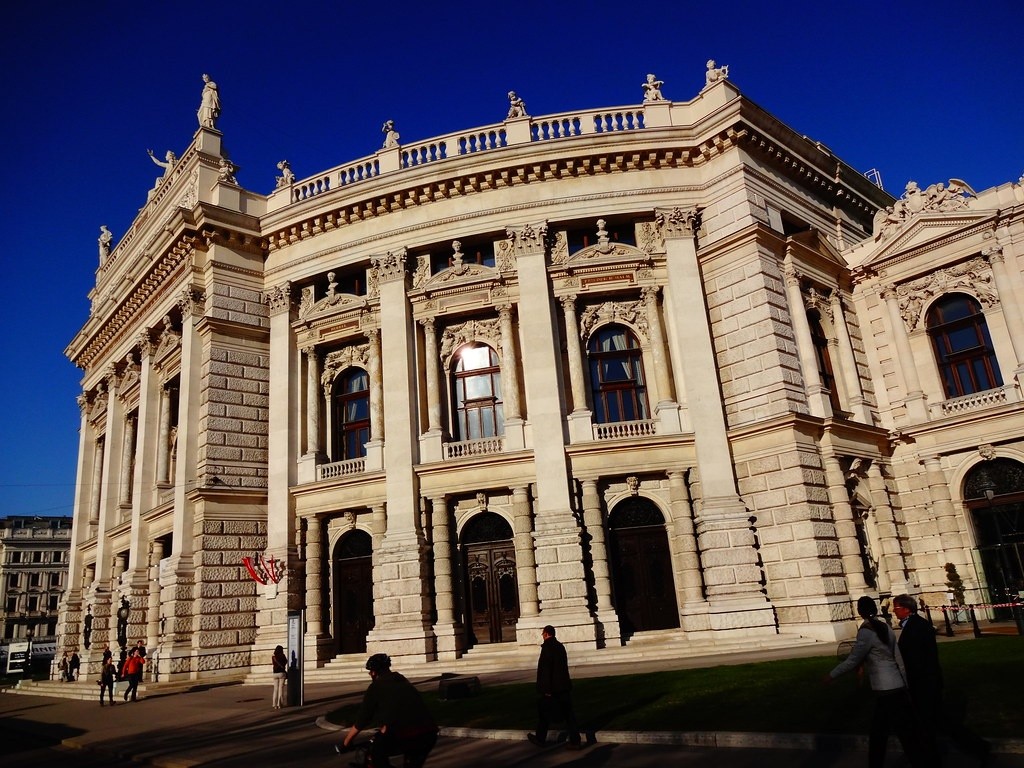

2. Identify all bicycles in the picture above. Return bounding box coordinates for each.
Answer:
[334,729,434,768]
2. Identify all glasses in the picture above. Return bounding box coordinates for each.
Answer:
[894,606,903,609]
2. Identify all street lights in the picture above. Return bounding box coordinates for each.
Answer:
[971,467,1024,638]
[25,620,32,680]
[117,597,129,683]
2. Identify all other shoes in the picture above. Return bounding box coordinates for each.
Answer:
[274,707,277,709]
[566,743,580,750]
[100,703,104,706]
[124,696,127,700]
[527,733,545,748]
[132,698,137,702]
[278,707,281,708]
[111,701,115,705]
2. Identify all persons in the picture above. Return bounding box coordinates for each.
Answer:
[327,271,339,290]
[380,120,400,150]
[439,330,454,363]
[57,651,69,682]
[581,306,600,345]
[894,593,992,768]
[196,73,221,129]
[595,219,608,238]
[701,59,728,90]
[526,624,581,751]
[271,645,288,710]
[138,147,180,213]
[65,651,80,682]
[641,73,666,102]
[873,198,917,244]
[217,158,240,187]
[98,225,113,268]
[98,656,118,707]
[505,91,528,118]
[275,160,296,189]
[822,595,931,768]
[334,653,439,768]
[924,178,979,212]
[96,646,112,683]
[319,356,341,396]
[452,240,464,260]
[128,640,147,682]
[121,647,145,702]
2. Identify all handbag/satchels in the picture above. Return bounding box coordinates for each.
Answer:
[285,671,288,679]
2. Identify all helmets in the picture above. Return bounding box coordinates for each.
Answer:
[366,654,391,671]
